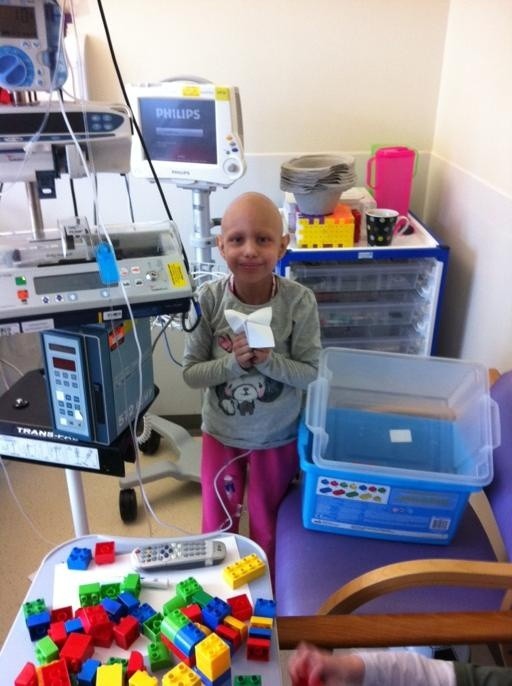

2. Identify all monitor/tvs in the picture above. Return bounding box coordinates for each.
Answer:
[127,85,244,190]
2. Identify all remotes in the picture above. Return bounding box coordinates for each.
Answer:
[135,539,227,568]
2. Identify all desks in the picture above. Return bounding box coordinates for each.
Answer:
[0,529,281,685]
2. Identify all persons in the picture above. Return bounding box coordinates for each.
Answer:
[287,639,512,686]
[178,190,323,594]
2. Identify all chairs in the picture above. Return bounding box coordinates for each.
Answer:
[272,365,512,652]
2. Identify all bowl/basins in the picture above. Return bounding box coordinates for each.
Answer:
[279,152,362,217]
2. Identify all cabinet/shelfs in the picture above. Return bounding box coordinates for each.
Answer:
[275,205,450,355]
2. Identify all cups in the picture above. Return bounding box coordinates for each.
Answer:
[363,208,408,245]
[93,241,121,284]
[366,145,416,224]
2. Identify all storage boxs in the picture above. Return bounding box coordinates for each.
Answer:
[303,345,503,485]
[296,404,483,547]
